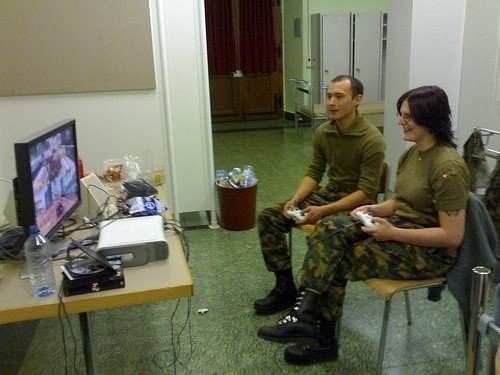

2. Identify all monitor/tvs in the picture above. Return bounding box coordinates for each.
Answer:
[11,116,82,246]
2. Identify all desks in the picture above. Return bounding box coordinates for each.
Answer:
[0,184,194,375]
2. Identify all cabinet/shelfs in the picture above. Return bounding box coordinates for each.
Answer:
[311,10,388,104]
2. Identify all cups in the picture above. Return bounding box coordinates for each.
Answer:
[105,159,121,181]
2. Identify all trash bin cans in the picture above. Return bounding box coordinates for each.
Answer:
[214,177,258,232]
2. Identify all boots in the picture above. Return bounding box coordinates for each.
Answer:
[255,269,297,316]
[257,289,324,343]
[285,317,338,364]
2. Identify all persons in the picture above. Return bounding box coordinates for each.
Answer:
[258,86,468,366]
[253,75,387,313]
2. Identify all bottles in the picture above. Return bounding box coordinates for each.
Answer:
[226,167,242,181]
[242,165,254,187]
[25,225,56,298]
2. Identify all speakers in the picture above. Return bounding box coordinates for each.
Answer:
[71,173,118,221]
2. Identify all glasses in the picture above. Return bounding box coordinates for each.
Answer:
[396,112,413,122]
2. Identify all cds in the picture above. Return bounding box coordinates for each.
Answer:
[69,260,106,275]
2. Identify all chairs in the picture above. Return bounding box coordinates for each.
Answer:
[288,160,481,375]
